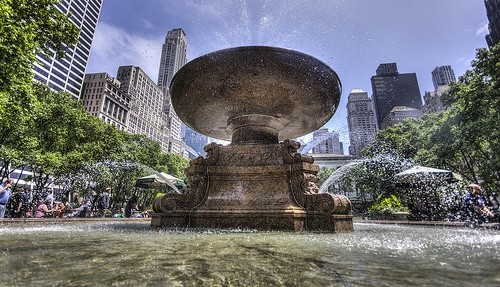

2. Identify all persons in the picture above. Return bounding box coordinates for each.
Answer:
[0,178,151,218]
[463,184,495,223]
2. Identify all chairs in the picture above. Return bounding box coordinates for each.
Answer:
[8,199,100,218]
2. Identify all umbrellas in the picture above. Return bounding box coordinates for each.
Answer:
[393,165,462,209]
[133,172,186,188]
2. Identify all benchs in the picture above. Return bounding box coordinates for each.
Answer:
[407,206,499,222]
